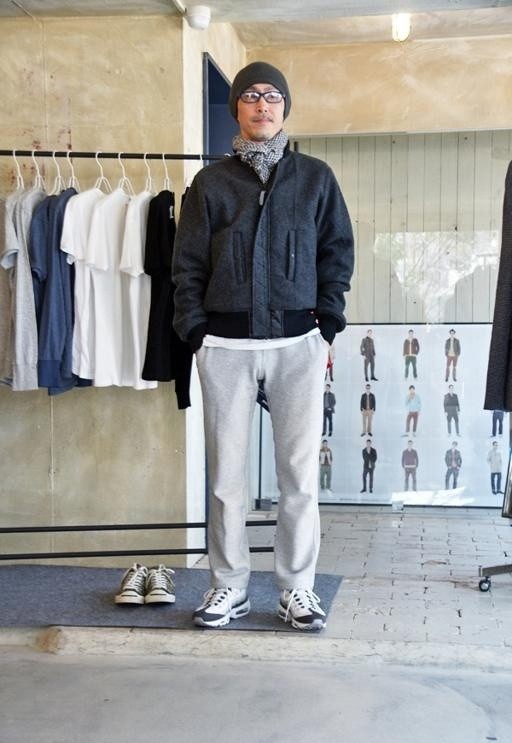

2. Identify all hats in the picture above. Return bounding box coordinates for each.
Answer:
[228,61,292,119]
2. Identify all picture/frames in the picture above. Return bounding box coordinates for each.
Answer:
[255,318,510,509]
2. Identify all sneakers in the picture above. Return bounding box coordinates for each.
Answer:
[192,588,251,628]
[114,562,149,605]
[144,563,176,604]
[276,588,327,631]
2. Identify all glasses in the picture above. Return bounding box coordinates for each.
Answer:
[240,91,284,104]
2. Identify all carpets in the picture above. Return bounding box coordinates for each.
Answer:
[1,562,348,638]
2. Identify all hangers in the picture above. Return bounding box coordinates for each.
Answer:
[9,148,206,193]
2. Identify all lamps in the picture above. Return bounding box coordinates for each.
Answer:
[388,10,415,42]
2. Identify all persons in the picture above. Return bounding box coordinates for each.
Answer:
[171,61,355,631]
[319,327,505,495]
